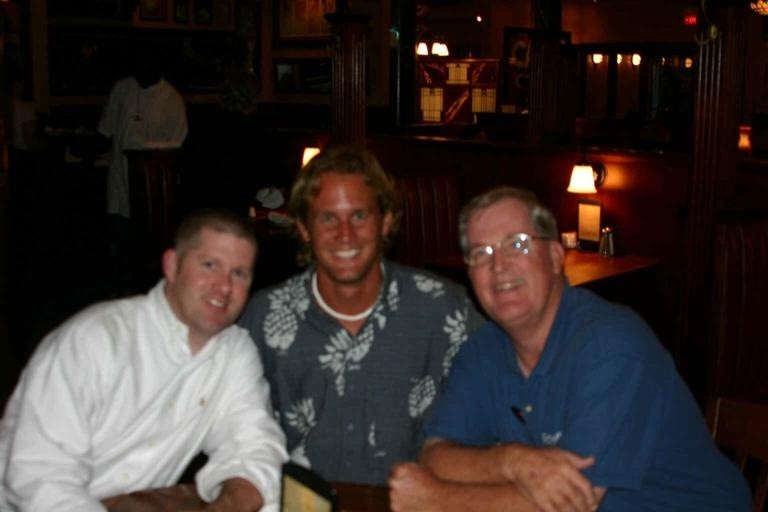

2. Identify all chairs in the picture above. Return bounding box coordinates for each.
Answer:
[703,389,768,510]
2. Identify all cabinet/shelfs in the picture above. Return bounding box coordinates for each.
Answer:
[564,40,698,157]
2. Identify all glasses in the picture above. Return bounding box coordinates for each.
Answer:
[462,233,551,269]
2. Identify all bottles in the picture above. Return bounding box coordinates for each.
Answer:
[607,227,614,255]
[599,228,609,256]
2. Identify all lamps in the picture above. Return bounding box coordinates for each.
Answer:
[414,38,448,61]
[566,163,608,198]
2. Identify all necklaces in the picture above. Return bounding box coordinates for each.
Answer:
[310,261,386,321]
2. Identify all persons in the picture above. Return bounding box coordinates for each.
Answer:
[94,40,193,224]
[388,186,754,512]
[0,214,291,512]
[236,147,484,488]
